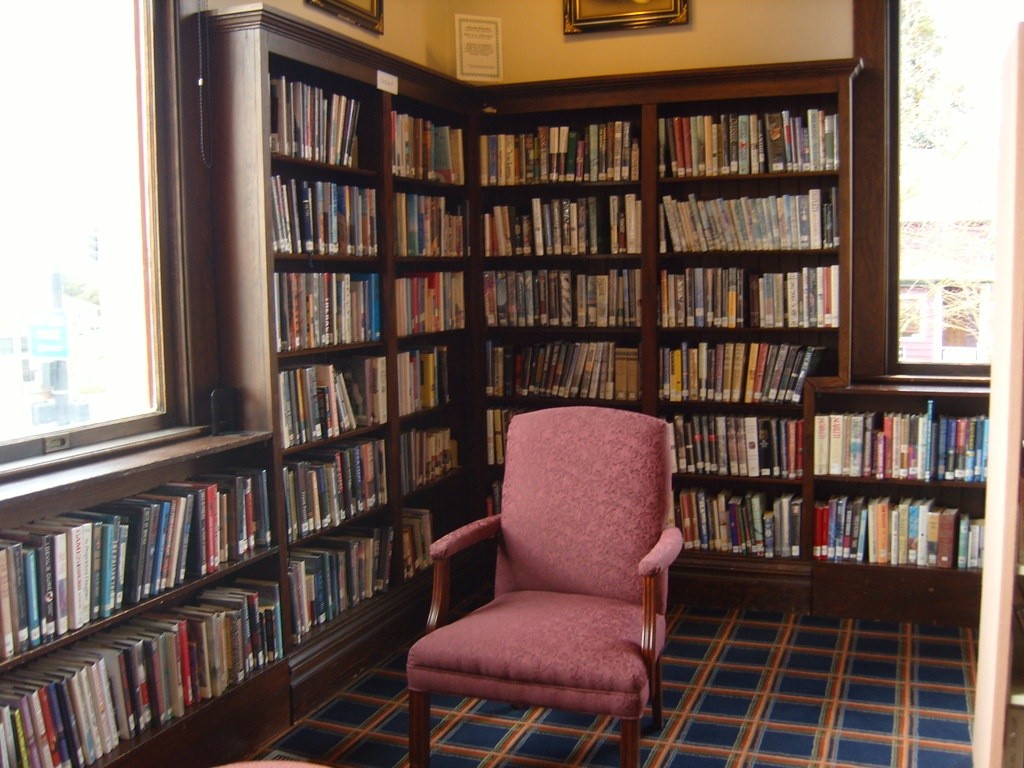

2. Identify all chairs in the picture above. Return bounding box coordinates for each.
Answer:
[406,406,684,768]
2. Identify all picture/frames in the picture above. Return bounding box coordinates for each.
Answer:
[305,0,384,35]
[563,0,689,35]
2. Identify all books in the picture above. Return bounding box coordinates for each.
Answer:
[270,75,988,645]
[0,467,285,768]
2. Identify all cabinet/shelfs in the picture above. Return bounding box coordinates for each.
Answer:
[0,3,1024,768]
